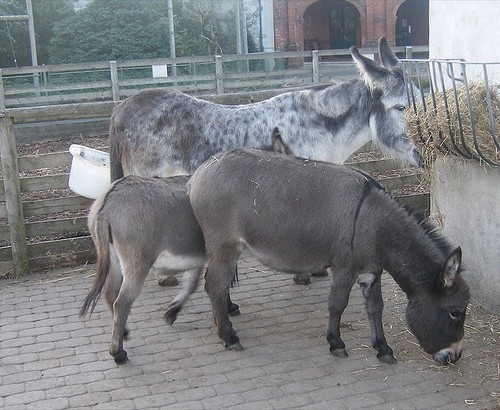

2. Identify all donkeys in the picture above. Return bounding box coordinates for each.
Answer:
[80,173,240,364]
[108,37,422,286]
[185,146,469,364]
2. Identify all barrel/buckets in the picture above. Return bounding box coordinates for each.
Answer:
[67,142,114,201]
